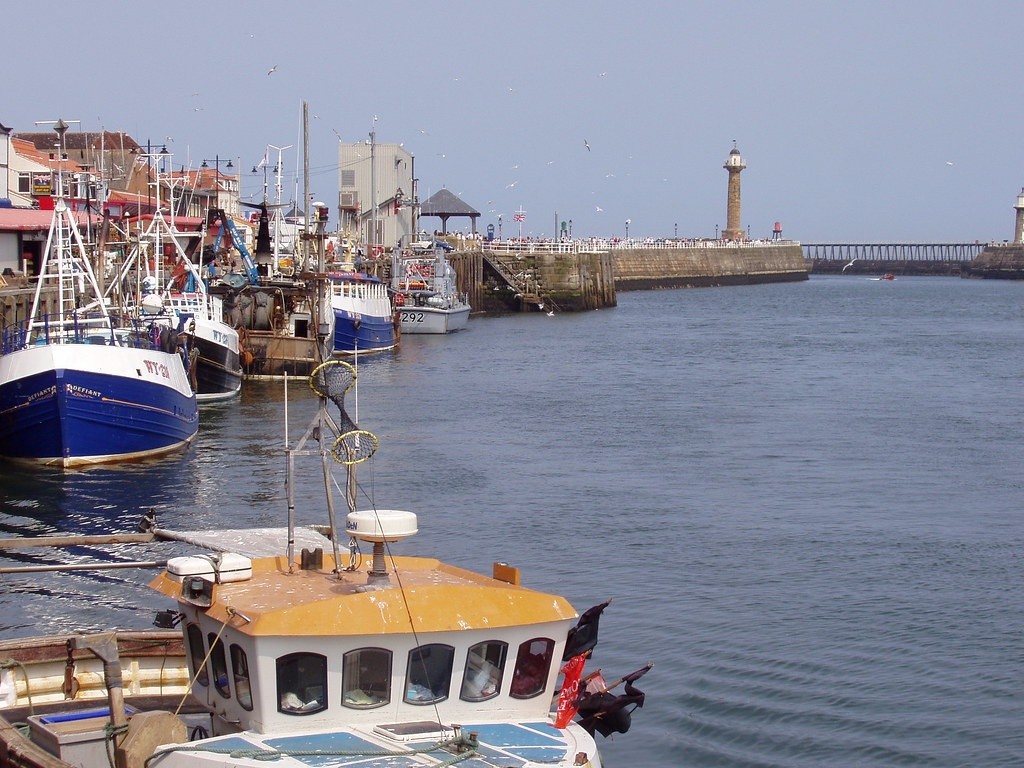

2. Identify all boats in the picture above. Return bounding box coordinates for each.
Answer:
[0,342,655,768]
[0,99,470,466]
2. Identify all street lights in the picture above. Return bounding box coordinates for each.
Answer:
[251,166,278,208]
[498,218,502,242]
[128,139,169,214]
[674,223,678,240]
[715,224,718,240]
[569,220,572,240]
[200,155,234,209]
